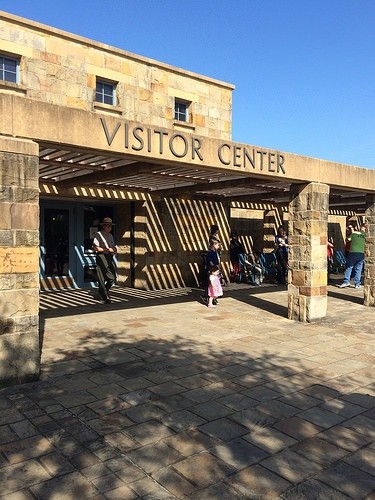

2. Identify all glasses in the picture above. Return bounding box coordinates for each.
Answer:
[103,224,112,228]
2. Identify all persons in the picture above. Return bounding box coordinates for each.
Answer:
[200,224,366,304]
[93,218,117,304]
[208,268,223,308]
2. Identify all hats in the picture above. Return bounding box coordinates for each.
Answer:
[99,218,115,225]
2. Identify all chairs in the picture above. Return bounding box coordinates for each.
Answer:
[335,251,347,272]
[238,253,288,283]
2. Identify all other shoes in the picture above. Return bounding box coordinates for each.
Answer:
[199,293,208,305]
[207,303,216,308]
[339,282,350,288]
[355,285,361,289]
[213,298,219,305]
[104,300,111,304]
